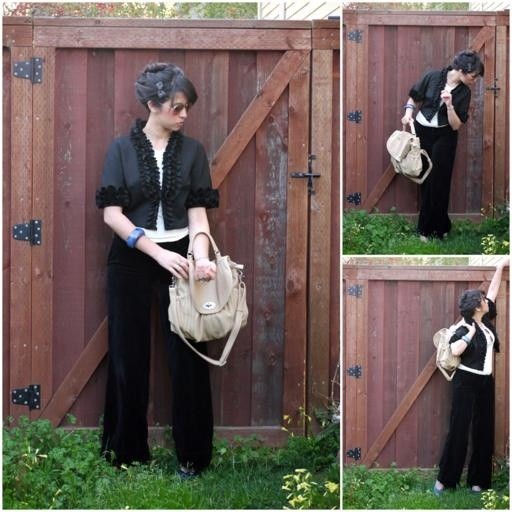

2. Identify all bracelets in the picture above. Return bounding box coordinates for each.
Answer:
[404,103,415,111]
[462,335,471,345]
[125,227,144,248]
[447,105,453,110]
[496,265,502,269]
[195,255,209,261]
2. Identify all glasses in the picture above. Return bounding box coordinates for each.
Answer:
[152,95,192,115]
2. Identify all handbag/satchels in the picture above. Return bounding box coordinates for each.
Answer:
[386,129,423,177]
[433,325,462,372]
[168,255,249,344]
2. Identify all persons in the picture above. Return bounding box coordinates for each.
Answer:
[95,63,220,482]
[401,50,485,243]
[434,259,509,496]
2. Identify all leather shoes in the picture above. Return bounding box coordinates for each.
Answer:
[434,485,444,495]
[471,489,482,495]
[176,463,198,480]
